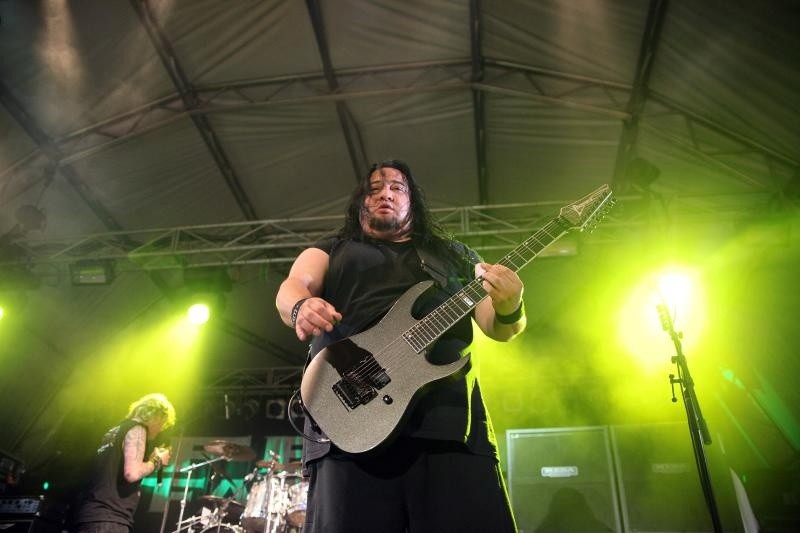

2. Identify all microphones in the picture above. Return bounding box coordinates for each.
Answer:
[269,450,281,460]
[156,448,166,486]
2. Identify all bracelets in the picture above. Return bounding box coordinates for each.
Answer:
[291,297,309,329]
[496,300,525,324]
[149,456,162,474]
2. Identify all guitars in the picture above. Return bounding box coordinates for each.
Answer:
[300,185,616,455]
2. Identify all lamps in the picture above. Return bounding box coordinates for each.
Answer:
[68,259,115,286]
[15,205,46,230]
[187,292,226,319]
[216,398,304,419]
[535,233,579,259]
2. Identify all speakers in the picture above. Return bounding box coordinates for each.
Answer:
[0,519,54,533]
[504,420,747,533]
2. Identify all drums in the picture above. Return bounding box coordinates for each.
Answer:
[200,523,246,533]
[239,478,290,533]
[288,481,309,528]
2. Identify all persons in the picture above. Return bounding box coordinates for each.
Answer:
[276,160,527,533]
[74,394,177,533]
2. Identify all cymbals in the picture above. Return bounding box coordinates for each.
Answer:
[289,460,302,466]
[256,460,287,471]
[203,440,254,461]
[195,495,245,507]
